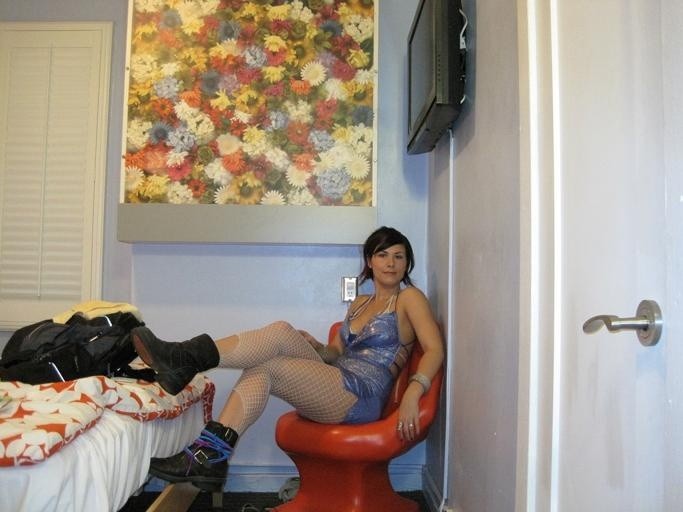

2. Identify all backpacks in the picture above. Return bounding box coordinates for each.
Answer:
[0,311,146,385]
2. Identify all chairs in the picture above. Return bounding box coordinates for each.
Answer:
[269,321,443,512]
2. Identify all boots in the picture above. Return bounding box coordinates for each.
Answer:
[148,418,239,493]
[130,325,221,398]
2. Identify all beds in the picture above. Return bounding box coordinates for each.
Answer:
[0,362,223,512]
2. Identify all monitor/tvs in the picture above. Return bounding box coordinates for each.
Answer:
[401,0,465,156]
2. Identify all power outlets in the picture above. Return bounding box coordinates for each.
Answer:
[342,277,359,302]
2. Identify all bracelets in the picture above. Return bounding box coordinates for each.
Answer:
[409,372,433,394]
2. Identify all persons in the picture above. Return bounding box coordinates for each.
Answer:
[131,225,445,493]
